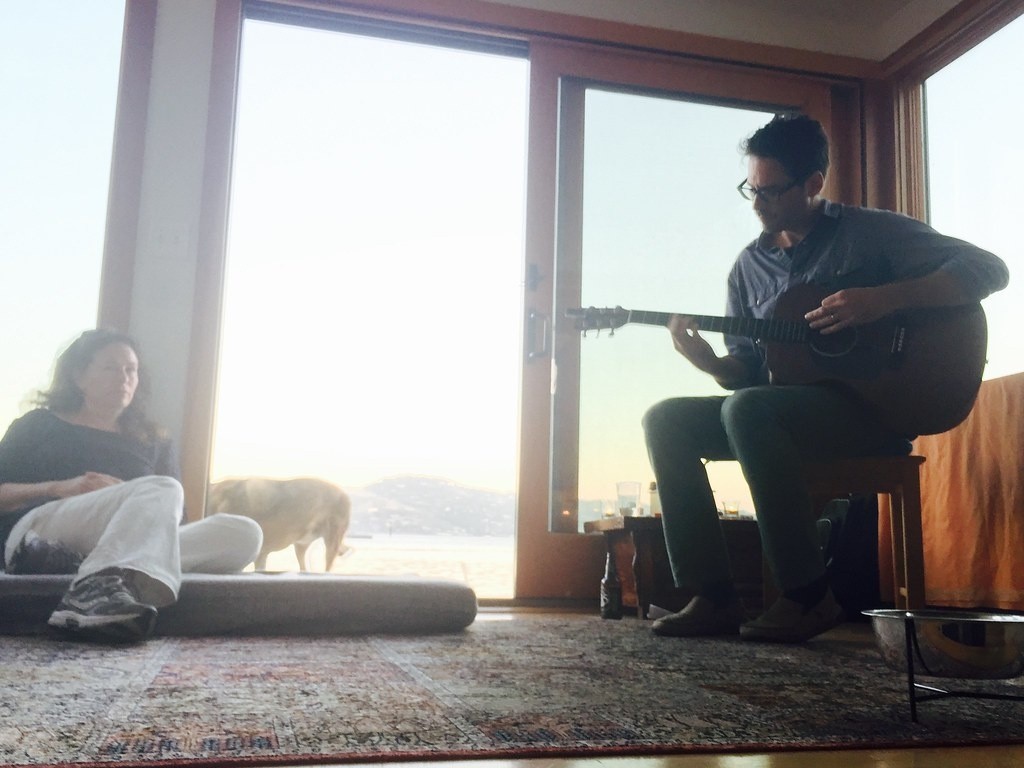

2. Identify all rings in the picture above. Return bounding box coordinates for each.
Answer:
[831,315,836,323]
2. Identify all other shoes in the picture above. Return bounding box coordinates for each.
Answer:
[738,597,836,643]
[653,596,756,637]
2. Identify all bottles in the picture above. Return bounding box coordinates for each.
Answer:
[648,481,659,517]
[600,551,623,619]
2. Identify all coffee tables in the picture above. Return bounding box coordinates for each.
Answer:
[582,512,767,622]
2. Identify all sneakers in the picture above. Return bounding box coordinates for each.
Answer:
[7,530,86,573]
[48,568,157,642]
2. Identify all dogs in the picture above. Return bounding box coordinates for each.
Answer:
[205,479,350,571]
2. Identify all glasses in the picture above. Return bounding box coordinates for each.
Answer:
[737,177,804,203]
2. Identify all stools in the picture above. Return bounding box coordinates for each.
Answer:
[796,454,929,608]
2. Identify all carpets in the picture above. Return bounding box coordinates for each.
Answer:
[0,613,1024,766]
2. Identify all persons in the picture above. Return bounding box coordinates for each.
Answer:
[643,112,1010,642]
[0,327,264,643]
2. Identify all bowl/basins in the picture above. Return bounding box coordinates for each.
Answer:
[864,607,1024,680]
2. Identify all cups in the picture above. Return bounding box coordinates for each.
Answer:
[722,500,741,517]
[615,482,641,516]
[601,499,616,518]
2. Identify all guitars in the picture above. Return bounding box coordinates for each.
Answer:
[547,300,989,437]
